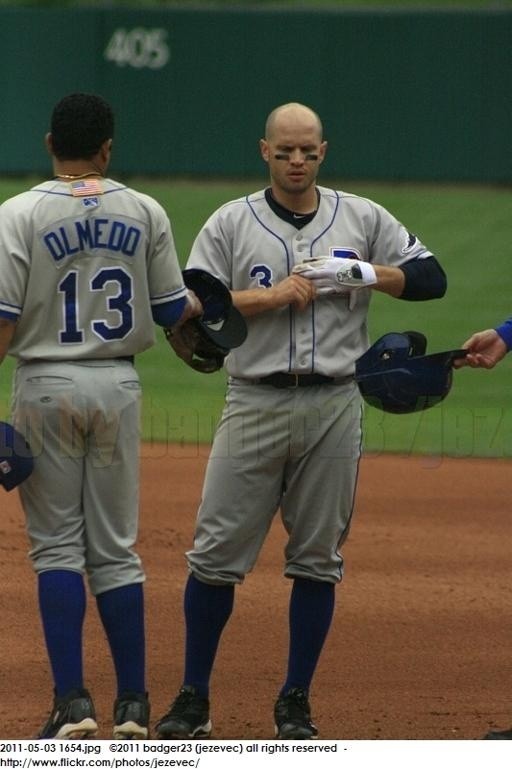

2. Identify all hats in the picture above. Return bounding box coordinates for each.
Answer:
[181,269,247,349]
[0,422,34,492]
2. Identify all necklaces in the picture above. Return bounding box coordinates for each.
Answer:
[56,171,100,179]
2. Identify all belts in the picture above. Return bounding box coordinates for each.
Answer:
[261,370,332,392]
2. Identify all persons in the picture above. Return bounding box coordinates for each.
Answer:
[452,314,512,370]
[154,101,446,740]
[0,93,203,739]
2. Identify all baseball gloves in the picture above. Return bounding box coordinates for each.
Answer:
[163,317,231,372]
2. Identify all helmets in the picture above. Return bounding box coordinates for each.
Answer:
[352,331,468,414]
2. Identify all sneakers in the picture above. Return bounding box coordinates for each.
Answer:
[40,686,98,740]
[113,688,150,740]
[271,688,319,739]
[155,685,212,739]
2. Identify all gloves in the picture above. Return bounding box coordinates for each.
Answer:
[293,255,376,310]
